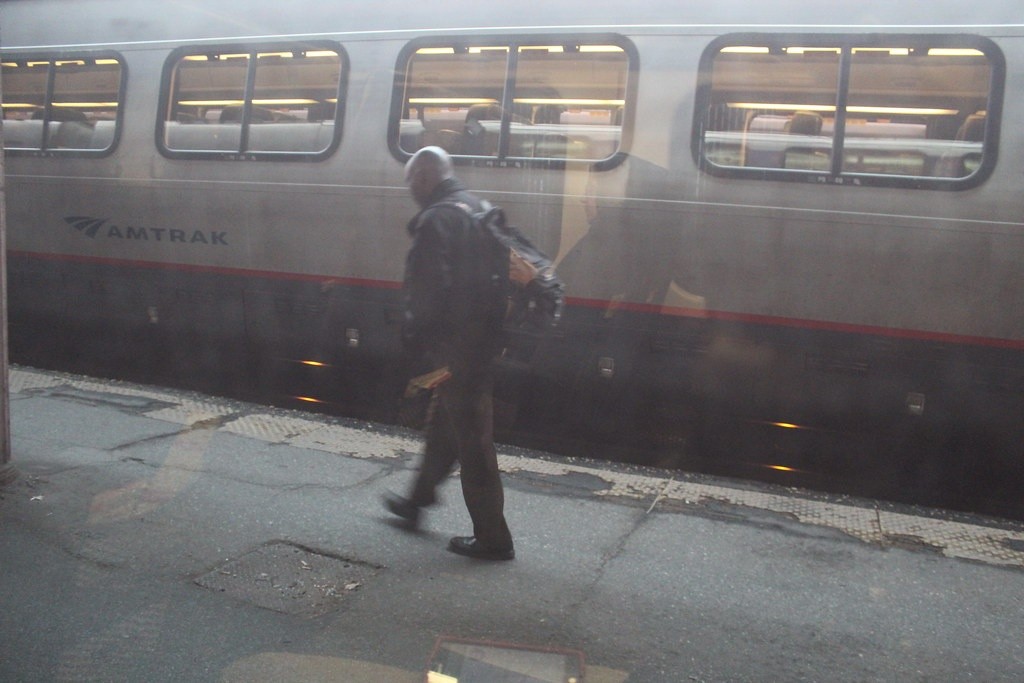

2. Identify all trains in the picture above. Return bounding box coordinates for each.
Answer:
[0,1,1023,470]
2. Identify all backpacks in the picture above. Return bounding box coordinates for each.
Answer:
[427,195,564,348]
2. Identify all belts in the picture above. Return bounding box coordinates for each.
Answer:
[451,536,515,560]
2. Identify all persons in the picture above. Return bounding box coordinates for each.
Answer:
[382,146,515,560]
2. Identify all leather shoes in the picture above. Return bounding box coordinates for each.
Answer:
[383,488,420,519]
